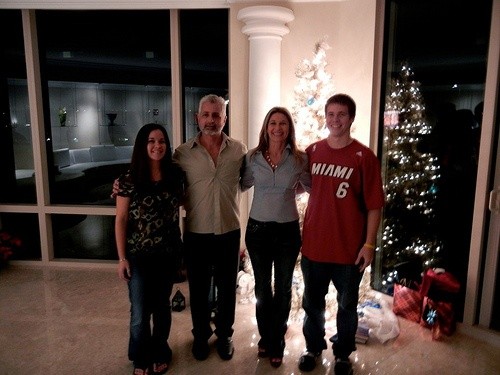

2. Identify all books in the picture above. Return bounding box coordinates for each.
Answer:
[355,325,369,344]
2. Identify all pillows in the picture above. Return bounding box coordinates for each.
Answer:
[90,146,116,161]
[55,148,71,168]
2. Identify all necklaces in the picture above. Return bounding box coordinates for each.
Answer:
[265,148,278,169]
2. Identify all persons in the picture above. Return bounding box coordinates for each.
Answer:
[295,93,384,375]
[115,123,188,375]
[240,107,311,369]
[111,94,250,361]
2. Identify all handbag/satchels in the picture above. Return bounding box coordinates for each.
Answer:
[392,279,423,324]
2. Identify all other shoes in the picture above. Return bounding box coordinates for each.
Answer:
[217,336,234,360]
[132,367,149,375]
[334,357,353,375]
[258,347,269,357]
[299,352,322,372]
[151,348,172,375]
[270,355,282,368]
[192,338,209,361]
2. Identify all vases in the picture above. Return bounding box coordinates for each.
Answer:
[59,107,67,127]
[107,113,117,126]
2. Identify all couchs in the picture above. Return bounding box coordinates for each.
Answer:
[54,145,135,204]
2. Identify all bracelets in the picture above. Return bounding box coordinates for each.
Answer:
[364,243,375,249]
[118,257,127,263]
